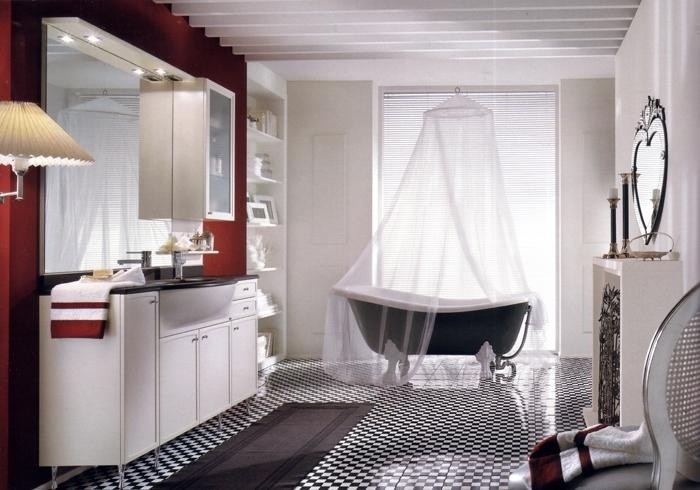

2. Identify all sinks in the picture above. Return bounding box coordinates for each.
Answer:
[160,279,237,328]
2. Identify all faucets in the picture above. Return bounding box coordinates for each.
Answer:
[172,249,220,280]
[117,250,152,268]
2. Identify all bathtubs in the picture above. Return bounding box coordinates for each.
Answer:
[333,284,539,357]
